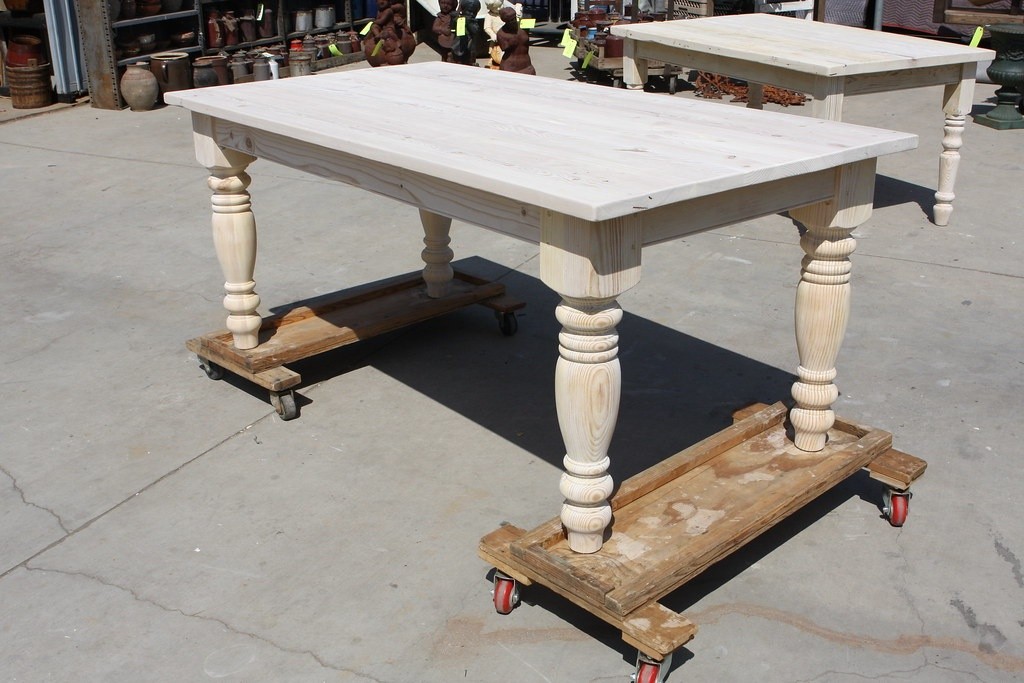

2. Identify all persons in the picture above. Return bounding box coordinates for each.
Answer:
[363,0,536,75]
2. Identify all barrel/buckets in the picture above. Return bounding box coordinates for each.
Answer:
[313,7,333,29]
[288,55,311,77]
[5,62,52,109]
[290,8,313,32]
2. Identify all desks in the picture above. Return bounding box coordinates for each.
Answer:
[611,14,997,225]
[163,60,920,553]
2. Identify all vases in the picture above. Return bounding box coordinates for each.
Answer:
[120,62,159,112]
[149,53,191,105]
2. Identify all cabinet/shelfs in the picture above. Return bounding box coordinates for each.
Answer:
[73,0,410,110]
